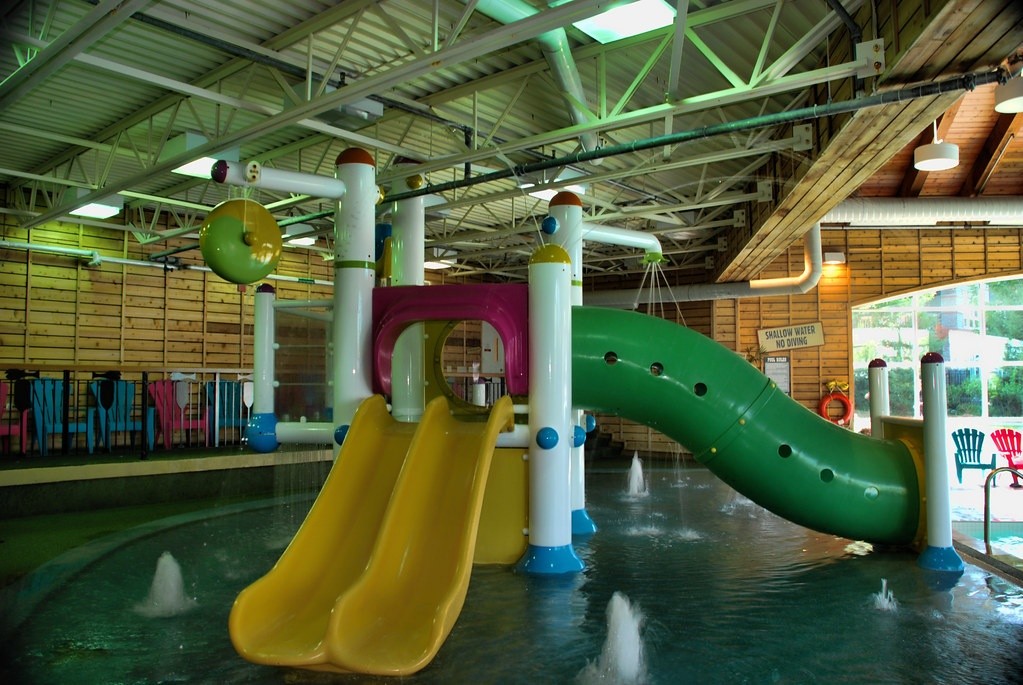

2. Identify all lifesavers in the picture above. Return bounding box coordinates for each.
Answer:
[819,393,852,426]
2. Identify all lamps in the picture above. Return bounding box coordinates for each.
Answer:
[518,163,588,200]
[61,186,125,218]
[547,0,678,45]
[285,83,384,128]
[423,242,458,268]
[823,252,845,263]
[913,119,960,171]
[280,224,319,247]
[656,209,710,239]
[995,74,1023,113]
[160,132,240,178]
[423,195,450,220]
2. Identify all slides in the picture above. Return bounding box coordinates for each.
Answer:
[571,305,920,546]
[228,393,514,676]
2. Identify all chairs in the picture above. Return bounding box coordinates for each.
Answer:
[0,382,30,454]
[990,429,1023,487]
[952,427,996,486]
[27,377,93,456]
[149,380,208,452]
[204,379,248,446]
[85,378,154,454]
